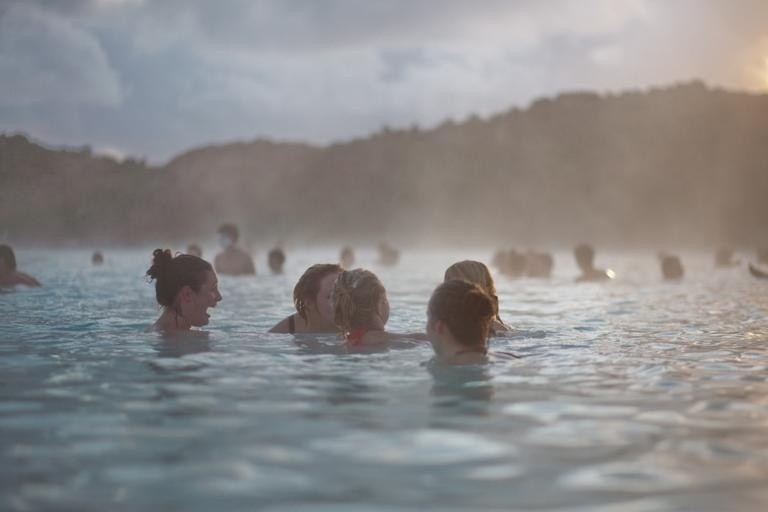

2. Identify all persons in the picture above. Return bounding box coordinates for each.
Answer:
[2,220,767,365]
